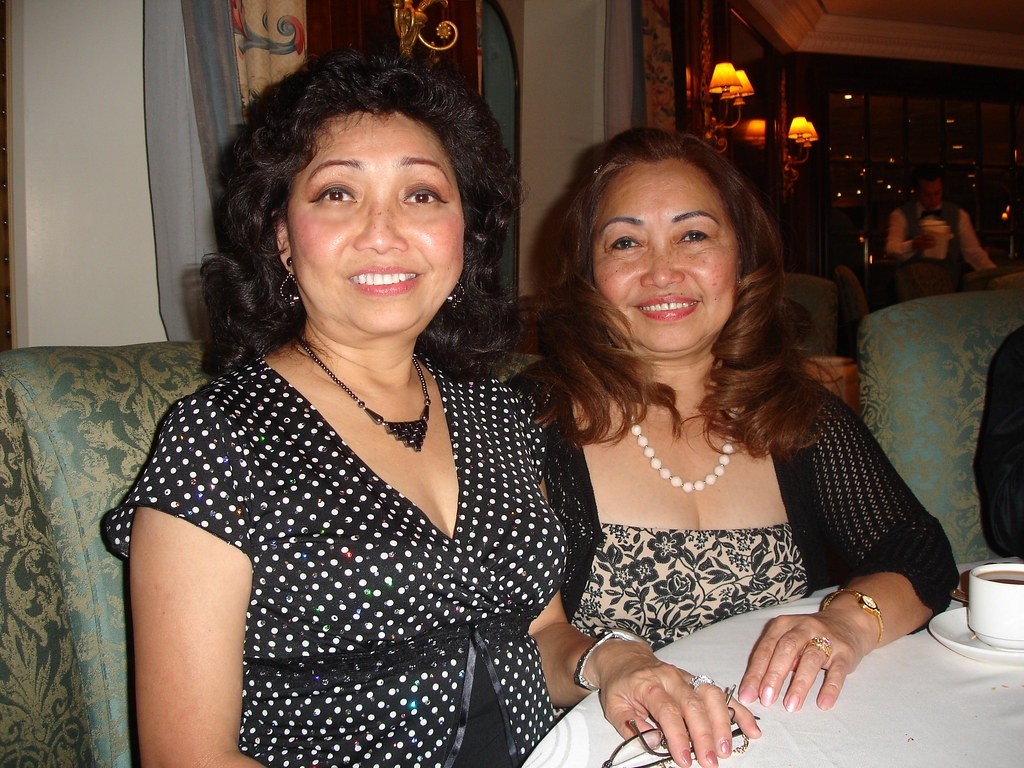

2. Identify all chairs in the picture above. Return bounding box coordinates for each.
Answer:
[0,340,261,767]
[773,263,1024,564]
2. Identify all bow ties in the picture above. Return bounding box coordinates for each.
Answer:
[921,208,943,218]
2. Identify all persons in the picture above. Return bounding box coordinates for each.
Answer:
[100,52,759,768]
[885,163,996,303]
[508,127,960,714]
[973,324,1024,558]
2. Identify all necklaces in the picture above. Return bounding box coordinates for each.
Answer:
[628,407,737,492]
[296,337,431,452]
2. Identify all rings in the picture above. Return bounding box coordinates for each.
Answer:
[690,675,716,689]
[802,637,832,665]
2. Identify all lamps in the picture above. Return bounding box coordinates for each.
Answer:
[703,63,755,154]
[782,116,819,182]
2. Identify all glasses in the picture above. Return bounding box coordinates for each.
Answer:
[601,683,744,768]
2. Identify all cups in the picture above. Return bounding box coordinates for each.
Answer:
[917,216,950,259]
[969,563,1024,651]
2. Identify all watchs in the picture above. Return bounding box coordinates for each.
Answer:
[574,628,648,692]
[821,588,884,643]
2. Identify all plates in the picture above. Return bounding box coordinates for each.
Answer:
[928,607,1024,664]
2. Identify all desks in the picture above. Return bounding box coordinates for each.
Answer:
[522,557,1024,768]
